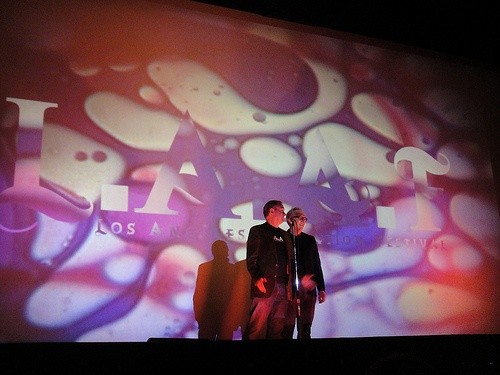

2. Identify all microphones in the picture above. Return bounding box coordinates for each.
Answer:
[286,215,295,221]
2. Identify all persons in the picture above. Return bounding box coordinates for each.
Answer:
[283,205,327,342]
[245,199,318,344]
